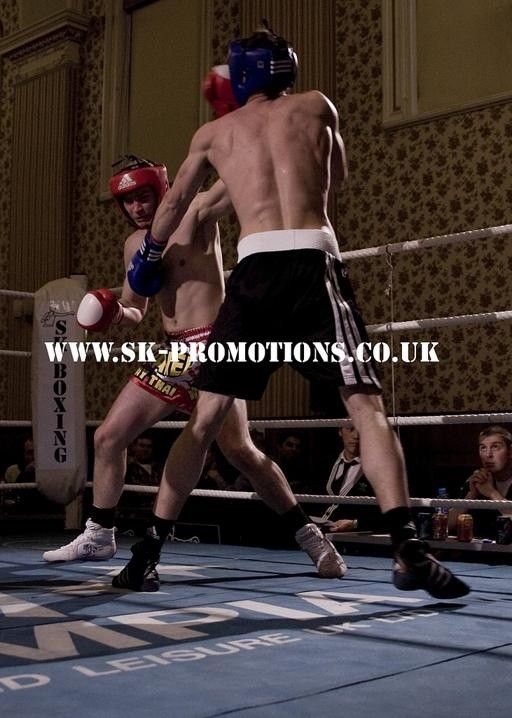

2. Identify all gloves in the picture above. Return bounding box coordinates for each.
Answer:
[126,232,167,297]
[203,64,236,118]
[76,288,125,336]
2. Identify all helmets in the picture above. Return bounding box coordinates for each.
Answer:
[227,30,300,107]
[109,154,171,230]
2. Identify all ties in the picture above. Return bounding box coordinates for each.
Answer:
[330,460,359,494]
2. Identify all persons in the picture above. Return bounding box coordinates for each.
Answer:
[0,421,313,551]
[308,427,381,533]
[111,28,472,599]
[447,425,512,543]
[40,57,351,584]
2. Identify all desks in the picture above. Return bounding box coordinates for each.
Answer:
[324,531,511,554]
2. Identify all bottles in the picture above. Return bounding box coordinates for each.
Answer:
[435,488,450,513]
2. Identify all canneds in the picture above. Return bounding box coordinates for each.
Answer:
[456,514,474,543]
[431,514,448,540]
[416,513,430,540]
[496,514,511,544]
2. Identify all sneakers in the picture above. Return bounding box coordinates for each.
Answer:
[41,519,117,562]
[111,552,161,592]
[391,538,471,600]
[293,523,347,579]
